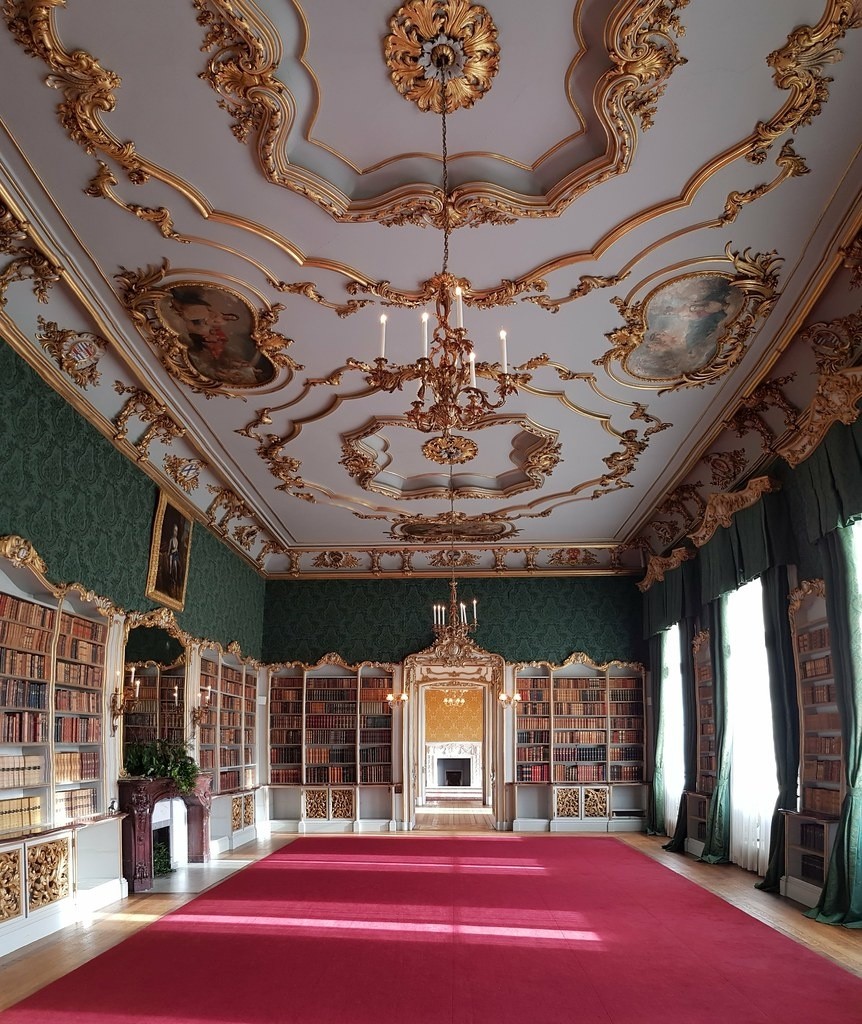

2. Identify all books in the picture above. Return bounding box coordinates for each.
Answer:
[1,592,106,842]
[199,659,257,794]
[799,626,841,817]
[123,676,185,774]
[698,664,715,793]
[270,677,393,785]
[517,678,644,781]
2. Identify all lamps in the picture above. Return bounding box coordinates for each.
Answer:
[499,691,522,709]
[368,1,521,433]
[441,690,470,709]
[385,692,408,710]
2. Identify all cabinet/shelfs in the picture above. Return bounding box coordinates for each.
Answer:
[188,636,403,860]
[506,652,653,833]
[123,655,185,762]
[777,578,846,909]
[684,630,718,858]
[0,533,128,960]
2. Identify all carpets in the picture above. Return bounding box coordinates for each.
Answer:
[0,837,862,1024]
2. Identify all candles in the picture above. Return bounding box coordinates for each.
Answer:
[422,436,480,638]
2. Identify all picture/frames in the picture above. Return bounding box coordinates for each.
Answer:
[144,490,195,613]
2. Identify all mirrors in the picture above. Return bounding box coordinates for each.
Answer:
[399,629,507,833]
[122,603,192,775]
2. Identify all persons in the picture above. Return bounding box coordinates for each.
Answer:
[166,524,179,580]
